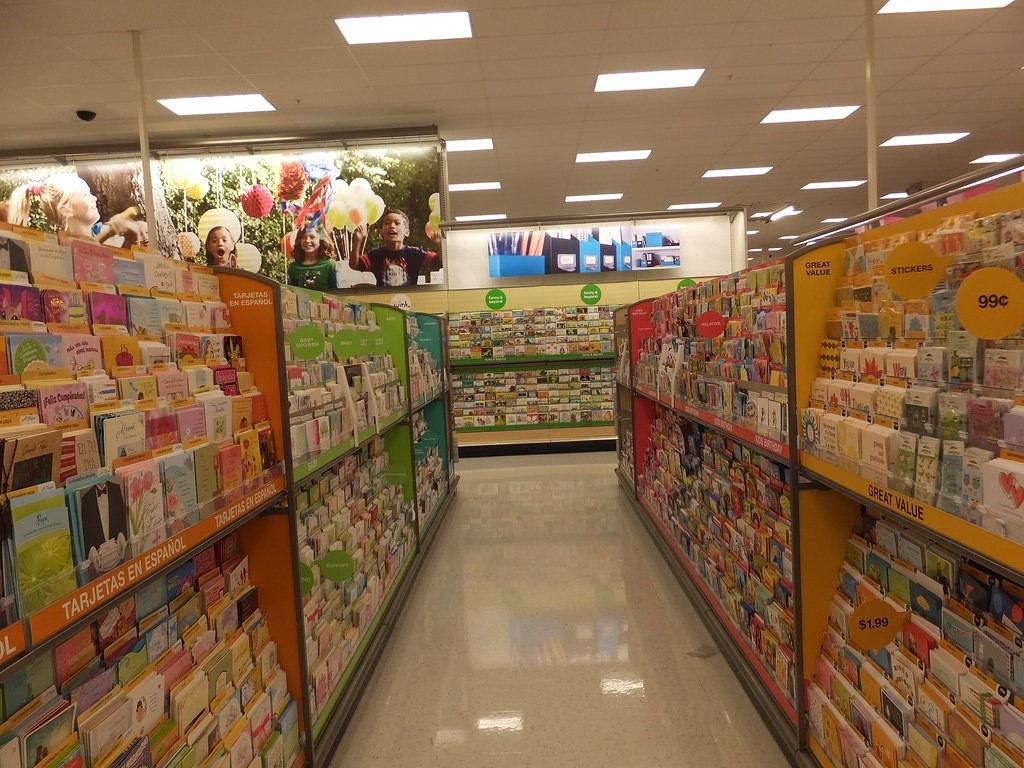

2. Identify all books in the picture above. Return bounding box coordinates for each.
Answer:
[635,204,1024,768]
[0,229,455,768]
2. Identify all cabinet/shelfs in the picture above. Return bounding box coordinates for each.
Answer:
[614,151,1024,768]
[0,220,461,768]
[433,304,624,458]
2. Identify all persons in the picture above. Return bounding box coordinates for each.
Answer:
[349,208,443,287]
[287,223,339,289]
[205,226,244,269]
[131,220,150,249]
[8,174,139,252]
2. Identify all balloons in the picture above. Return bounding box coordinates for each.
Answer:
[163,154,441,289]
